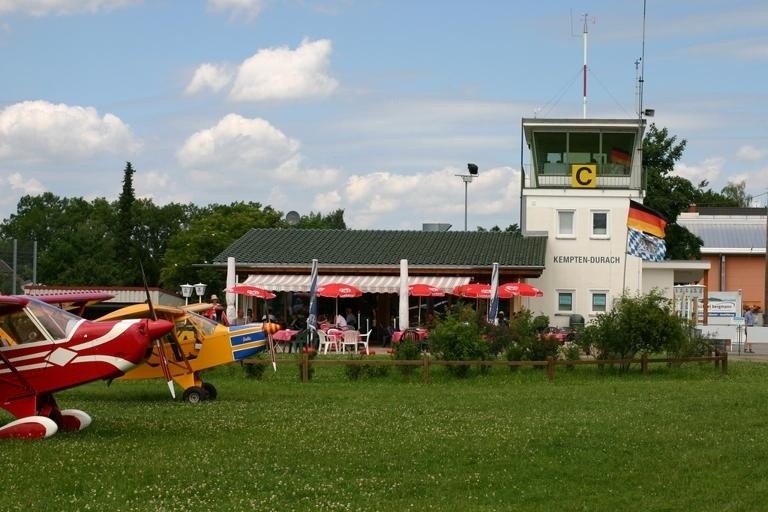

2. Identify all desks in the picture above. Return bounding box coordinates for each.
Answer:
[272,330,299,352]
[392,329,428,351]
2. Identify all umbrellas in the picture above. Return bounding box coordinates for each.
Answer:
[222,286,277,325]
[315,284,362,328]
[451,282,544,321]
[399,284,445,327]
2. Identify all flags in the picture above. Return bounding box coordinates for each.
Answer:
[626,229,668,263]
[626,199,668,239]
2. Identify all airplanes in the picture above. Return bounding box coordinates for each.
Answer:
[1,264,175,438]
[93,292,277,403]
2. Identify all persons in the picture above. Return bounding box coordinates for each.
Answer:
[317,306,357,328]
[743,308,755,354]
[290,313,302,330]
[423,313,437,330]
[261,307,276,324]
[207,295,223,319]
[246,308,258,323]
[497,311,510,328]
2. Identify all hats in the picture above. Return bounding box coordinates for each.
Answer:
[211,295,218,300]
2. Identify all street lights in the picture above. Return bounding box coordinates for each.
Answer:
[675,283,706,328]
[457,163,480,229]
[182,283,206,325]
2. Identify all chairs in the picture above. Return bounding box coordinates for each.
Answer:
[400,328,419,345]
[316,324,373,355]
